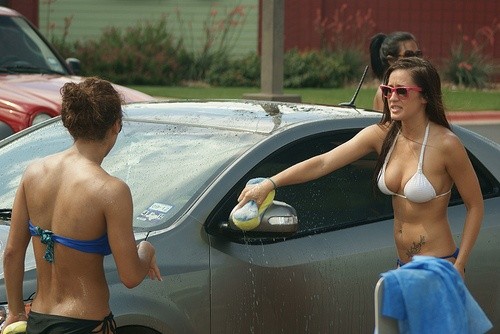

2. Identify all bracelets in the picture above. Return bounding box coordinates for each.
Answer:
[266,177,277,190]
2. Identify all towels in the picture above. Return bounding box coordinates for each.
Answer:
[379,254,493,334]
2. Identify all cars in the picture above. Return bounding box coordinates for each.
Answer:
[0,98,500,334]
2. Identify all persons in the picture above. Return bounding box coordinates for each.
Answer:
[367,32,421,116]
[1,74,163,334]
[232,54,484,281]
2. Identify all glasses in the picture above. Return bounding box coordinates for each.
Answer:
[394,50,424,60]
[379,84,425,100]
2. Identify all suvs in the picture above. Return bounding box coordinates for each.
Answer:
[0,5,159,143]
